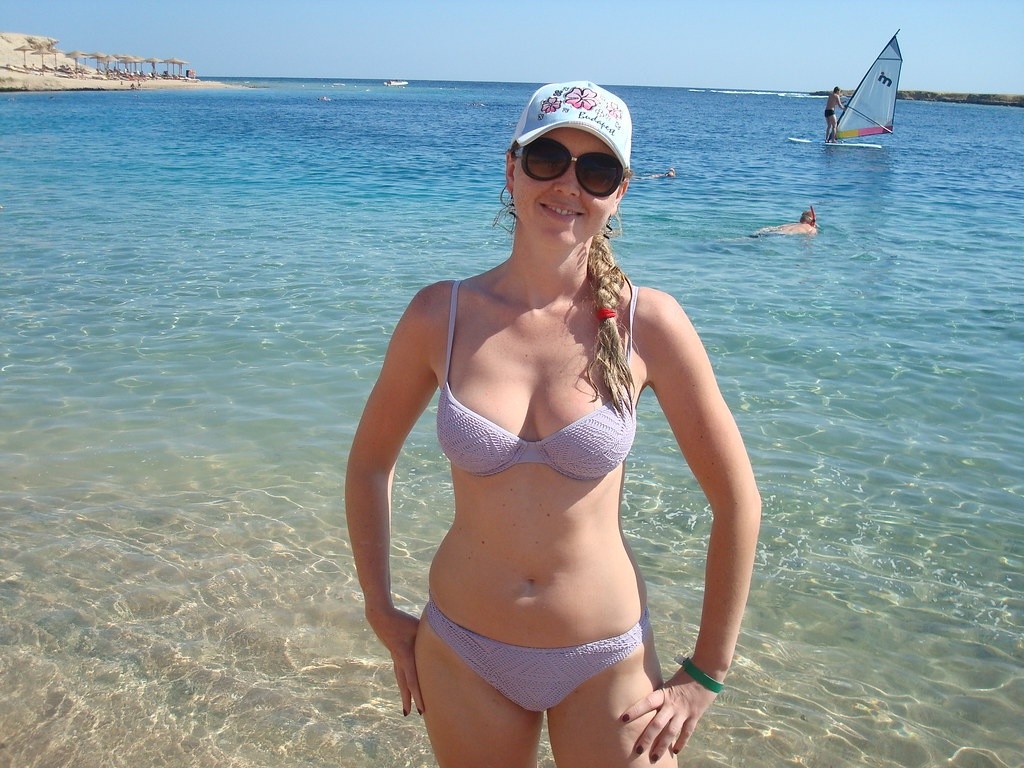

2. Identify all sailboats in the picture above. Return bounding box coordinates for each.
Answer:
[787,28,903,149]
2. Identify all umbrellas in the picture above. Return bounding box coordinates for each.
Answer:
[13,45,189,75]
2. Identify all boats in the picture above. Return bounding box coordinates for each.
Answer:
[384,81,409,86]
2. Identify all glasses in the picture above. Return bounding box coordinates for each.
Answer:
[511,137,624,197]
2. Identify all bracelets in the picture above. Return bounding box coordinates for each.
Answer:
[673,654,724,694]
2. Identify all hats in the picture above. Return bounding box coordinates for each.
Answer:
[511,80,632,169]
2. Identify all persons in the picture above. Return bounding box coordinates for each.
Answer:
[640,171,674,180]
[345,79,763,768]
[824,86,847,143]
[6,63,177,80]
[750,210,817,238]
